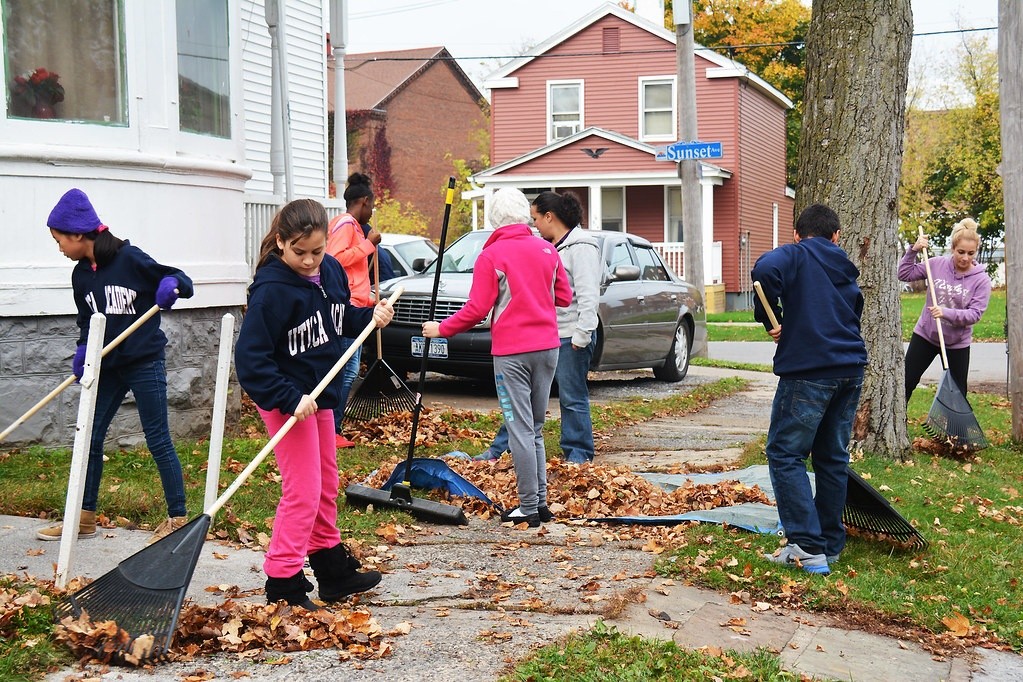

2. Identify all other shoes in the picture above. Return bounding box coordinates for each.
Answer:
[501,510,539,527]
[539,506,552,523]
[471,449,498,462]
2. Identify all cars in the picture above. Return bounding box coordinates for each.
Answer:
[378,234,439,278]
[366,229,708,390]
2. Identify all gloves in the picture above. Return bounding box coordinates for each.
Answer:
[72,343,86,384]
[156,278,179,312]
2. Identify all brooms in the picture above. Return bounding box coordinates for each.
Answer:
[750,280,922,548]
[53,285,411,661]
[342,227,426,422]
[0,284,179,438]
[341,178,468,526]
[916,222,989,453]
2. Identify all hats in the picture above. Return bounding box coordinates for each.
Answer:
[489,187,531,229]
[47,187,101,234]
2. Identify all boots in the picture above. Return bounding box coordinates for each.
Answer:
[265,572,331,614]
[308,542,381,601]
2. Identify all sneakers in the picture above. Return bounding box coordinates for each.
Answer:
[335,434,356,448]
[765,541,827,575]
[144,517,189,547]
[824,555,839,562]
[37,507,97,541]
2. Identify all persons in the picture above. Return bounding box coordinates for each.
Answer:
[750,205,868,576]
[322,172,394,450]
[36,187,194,548]
[233,197,394,612]
[470,190,601,465]
[897,217,991,409]
[421,186,572,528]
